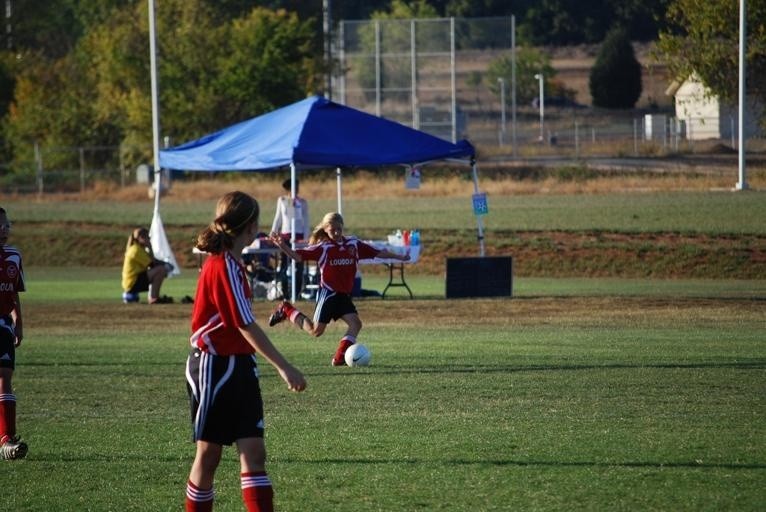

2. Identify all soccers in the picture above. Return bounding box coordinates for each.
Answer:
[123,293,139,304]
[344,344,370,367]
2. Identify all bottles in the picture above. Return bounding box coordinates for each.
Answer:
[395,227,420,245]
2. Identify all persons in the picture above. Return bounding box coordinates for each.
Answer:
[267,212,409,366]
[121,227,174,305]
[182,191,306,512]
[265,178,311,300]
[0,208,28,461]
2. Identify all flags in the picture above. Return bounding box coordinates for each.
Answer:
[144,211,181,277]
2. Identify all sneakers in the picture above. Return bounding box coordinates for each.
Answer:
[269,298,291,329]
[0,435,27,461]
[330,358,348,366]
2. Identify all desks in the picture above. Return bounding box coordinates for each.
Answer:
[192,239,421,303]
